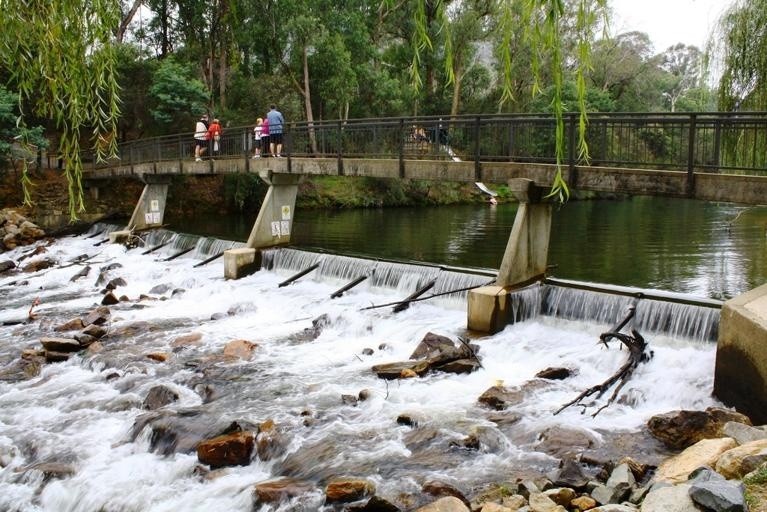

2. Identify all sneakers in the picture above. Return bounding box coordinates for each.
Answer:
[195,157,201,161]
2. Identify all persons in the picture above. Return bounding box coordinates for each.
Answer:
[261,119,271,158]
[252,117,264,159]
[202,117,224,159]
[193,114,210,163]
[266,103,285,158]
[409,124,427,144]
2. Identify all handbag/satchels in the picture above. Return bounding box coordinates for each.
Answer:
[214,131,221,140]
